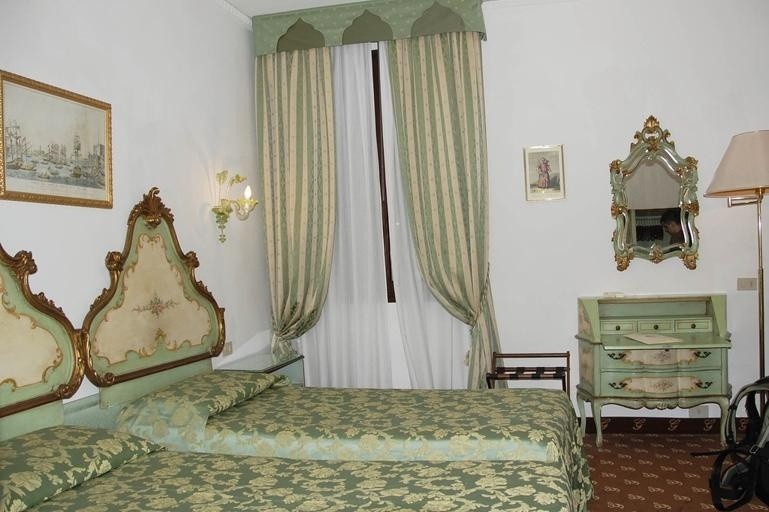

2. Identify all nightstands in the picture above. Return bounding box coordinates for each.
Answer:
[216,350,307,387]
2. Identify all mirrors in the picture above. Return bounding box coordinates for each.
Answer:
[608,112,702,273]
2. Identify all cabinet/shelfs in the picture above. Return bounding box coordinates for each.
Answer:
[574,291,735,449]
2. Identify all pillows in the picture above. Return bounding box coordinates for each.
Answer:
[0,424,169,512]
[115,369,292,436]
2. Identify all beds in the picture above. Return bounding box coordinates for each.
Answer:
[78,184,597,460]
[0,238,593,512]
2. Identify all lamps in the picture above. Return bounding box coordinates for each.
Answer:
[207,169,260,246]
[701,129,769,416]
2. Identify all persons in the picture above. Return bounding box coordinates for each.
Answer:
[537,157,551,188]
[660,211,685,245]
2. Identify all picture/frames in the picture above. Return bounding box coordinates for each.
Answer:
[522,144,568,203]
[0,68,116,212]
